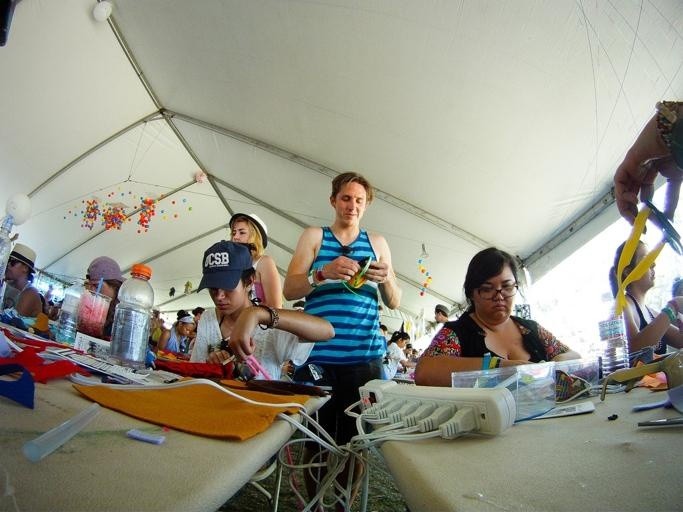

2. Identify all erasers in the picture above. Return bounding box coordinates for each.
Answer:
[126,430,165,444]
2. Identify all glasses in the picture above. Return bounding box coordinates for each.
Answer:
[478,283,519,299]
[8,258,21,266]
[343,245,354,257]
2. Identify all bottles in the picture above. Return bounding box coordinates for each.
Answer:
[595,291,629,388]
[109,261,155,372]
[56,293,81,348]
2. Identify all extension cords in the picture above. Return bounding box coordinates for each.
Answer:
[359,379,516,440]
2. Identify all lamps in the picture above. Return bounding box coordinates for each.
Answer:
[92,0,115,23]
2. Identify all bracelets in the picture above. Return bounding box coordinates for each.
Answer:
[667,299,678,312]
[660,305,675,326]
[482,350,502,386]
[314,270,325,282]
[255,301,280,330]
[653,98,682,174]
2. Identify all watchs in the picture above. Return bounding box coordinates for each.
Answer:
[305,268,317,289]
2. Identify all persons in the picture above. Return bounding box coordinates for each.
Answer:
[227,212,283,309]
[83,255,127,342]
[612,100,682,236]
[378,325,416,381]
[2,243,42,318]
[606,239,683,367]
[190,240,335,382]
[416,247,586,386]
[434,303,449,325]
[282,170,401,511]
[147,306,205,355]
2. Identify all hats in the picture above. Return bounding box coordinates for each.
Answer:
[436,305,450,316]
[86,255,128,283]
[230,212,268,248]
[196,240,253,293]
[10,243,37,274]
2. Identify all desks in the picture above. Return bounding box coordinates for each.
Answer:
[368,386,681,511]
[0,317,332,511]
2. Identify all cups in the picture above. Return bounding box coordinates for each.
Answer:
[75,289,112,340]
